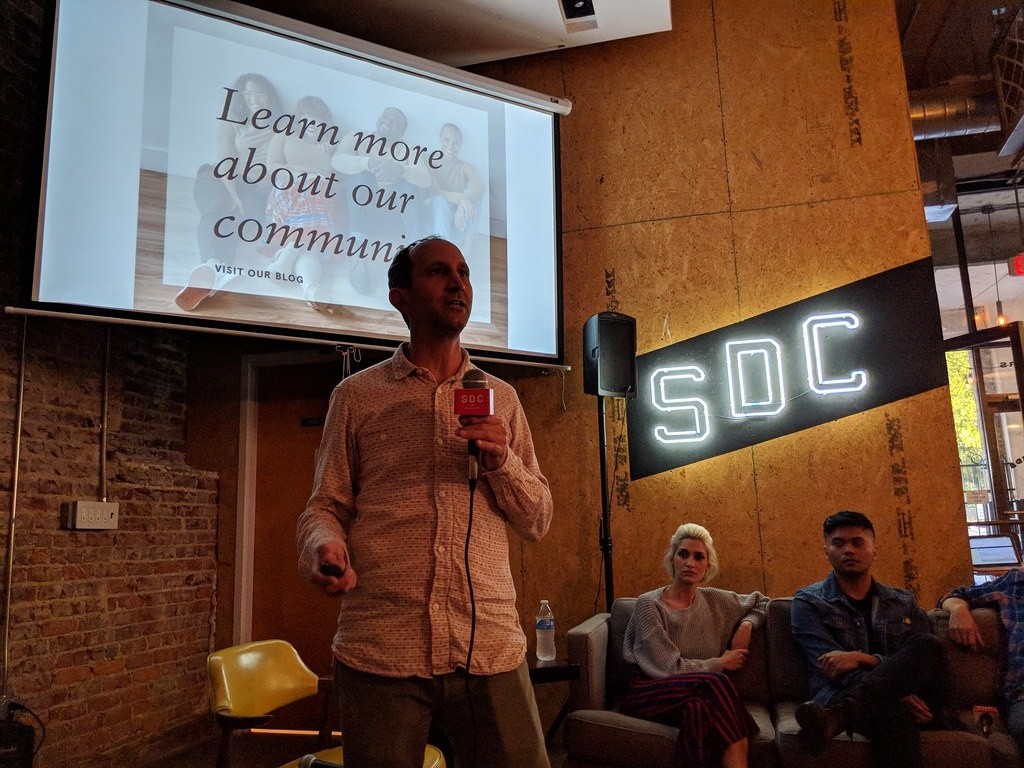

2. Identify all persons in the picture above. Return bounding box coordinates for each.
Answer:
[790,512,949,768]
[297,234,553,768]
[937,562,1024,759]
[624,524,771,768]
[176,73,486,317]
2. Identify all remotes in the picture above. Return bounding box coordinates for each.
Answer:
[319,561,344,579]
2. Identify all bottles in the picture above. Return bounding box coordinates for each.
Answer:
[536,599,555,662]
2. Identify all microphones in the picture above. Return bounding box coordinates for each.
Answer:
[980,713,993,737]
[454,369,494,491]
[297,753,344,768]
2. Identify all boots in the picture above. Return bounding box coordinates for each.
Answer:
[795,700,851,750]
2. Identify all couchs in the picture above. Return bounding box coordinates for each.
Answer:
[561,598,1022,767]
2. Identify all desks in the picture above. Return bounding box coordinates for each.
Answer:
[525,654,575,750]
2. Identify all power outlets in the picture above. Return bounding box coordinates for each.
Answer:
[74,500,119,530]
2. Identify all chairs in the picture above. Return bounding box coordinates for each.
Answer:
[204,639,448,768]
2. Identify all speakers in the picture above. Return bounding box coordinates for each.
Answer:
[0,717,33,768]
[582,311,639,398]
